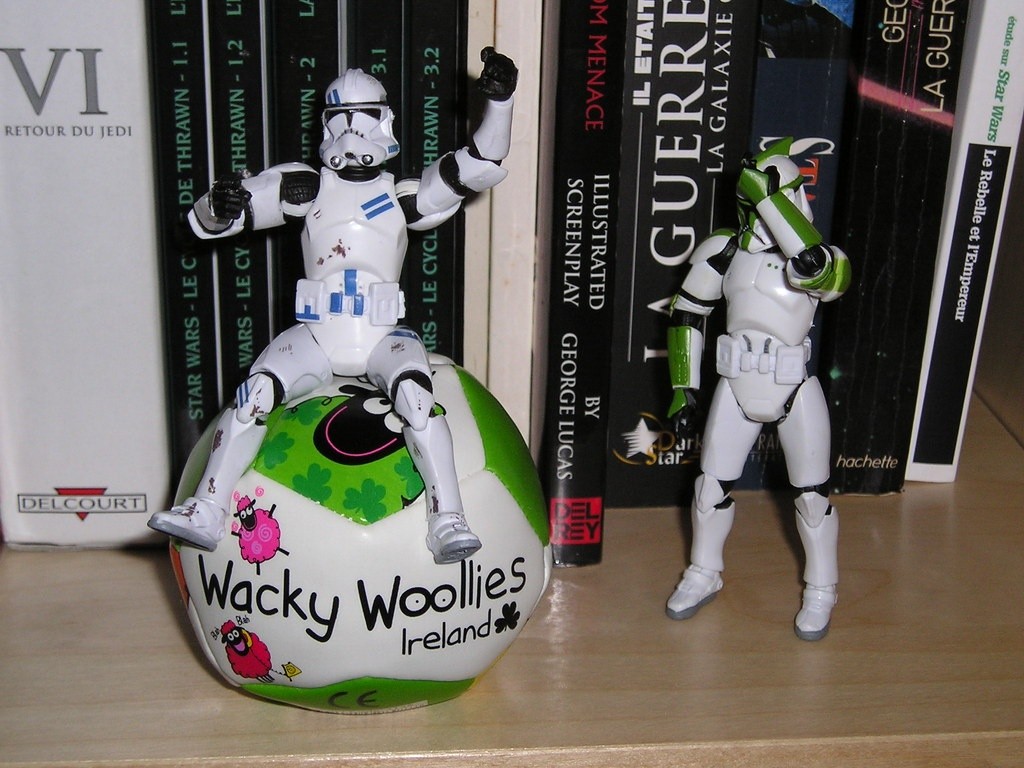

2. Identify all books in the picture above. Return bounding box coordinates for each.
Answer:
[0,0,1024,571]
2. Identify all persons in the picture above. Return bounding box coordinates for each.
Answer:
[142,47,521,566]
[662,135,851,641]
